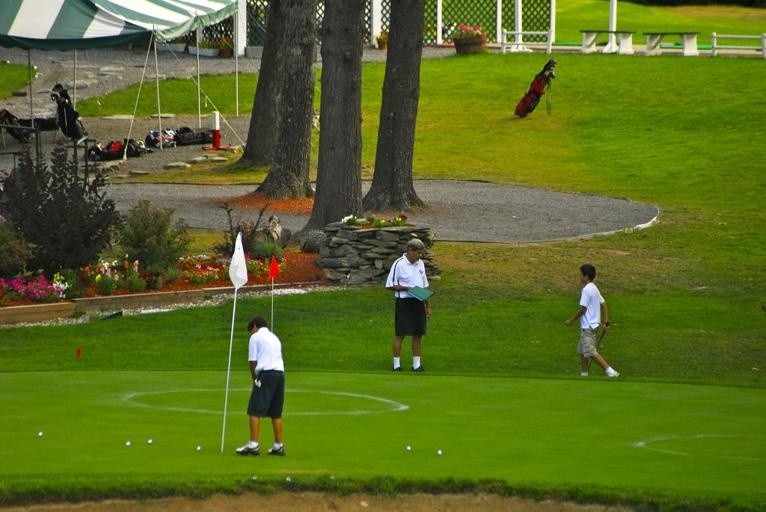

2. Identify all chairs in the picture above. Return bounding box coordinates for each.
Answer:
[268,446,285,456]
[412,367,424,371]
[235,444,260,456]
[393,366,402,371]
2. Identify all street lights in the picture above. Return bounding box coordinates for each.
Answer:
[146,127,221,148]
[88,139,154,161]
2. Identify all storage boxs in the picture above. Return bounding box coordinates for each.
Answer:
[642,31,701,56]
[579,30,636,54]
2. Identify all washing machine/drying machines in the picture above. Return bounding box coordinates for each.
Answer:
[540,61,557,80]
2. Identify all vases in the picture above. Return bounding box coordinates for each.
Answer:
[219,36,233,49]
[190,37,218,49]
[457,23,481,37]
[167,36,185,43]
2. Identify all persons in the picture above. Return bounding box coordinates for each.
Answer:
[385,239,432,371]
[563,264,620,377]
[235,316,286,455]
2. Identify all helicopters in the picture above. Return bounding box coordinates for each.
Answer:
[286,476,291,481]
[197,445,202,451]
[251,475,257,479]
[125,441,130,445]
[37,431,43,436]
[438,450,443,455]
[406,445,411,450]
[147,439,153,444]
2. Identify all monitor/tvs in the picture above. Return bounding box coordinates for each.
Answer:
[408,286,433,301]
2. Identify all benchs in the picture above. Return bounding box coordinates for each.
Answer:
[268,256,280,280]
[229,233,248,288]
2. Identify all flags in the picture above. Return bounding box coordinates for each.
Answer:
[453,32,487,55]
[156,43,187,52]
[223,48,233,57]
[188,45,220,57]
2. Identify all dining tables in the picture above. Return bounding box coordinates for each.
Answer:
[375,32,388,49]
